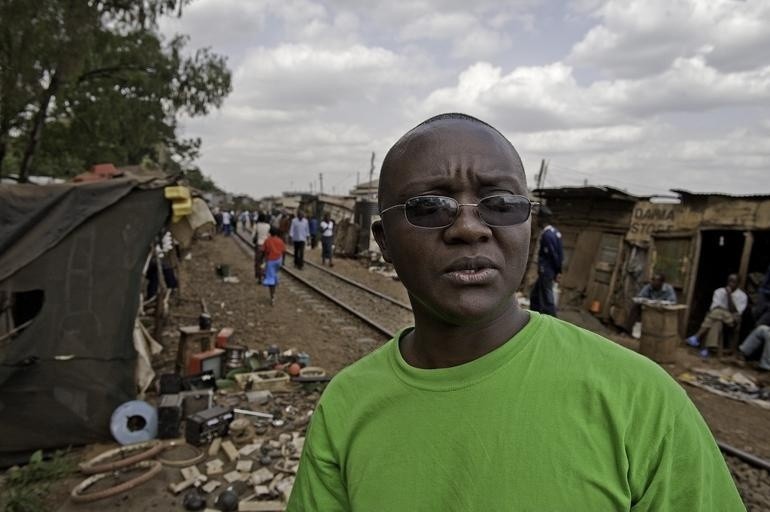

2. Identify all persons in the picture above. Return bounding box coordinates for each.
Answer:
[257,227,287,307]
[684,271,749,359]
[531,205,565,317]
[212,203,336,286]
[620,272,678,339]
[147,258,179,303]
[289,111,746,511]
[719,311,769,373]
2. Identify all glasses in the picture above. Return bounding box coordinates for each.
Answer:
[379,194,541,231]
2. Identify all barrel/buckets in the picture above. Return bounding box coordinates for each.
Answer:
[640,307,678,373]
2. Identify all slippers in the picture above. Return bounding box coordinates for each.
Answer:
[684,334,708,358]
